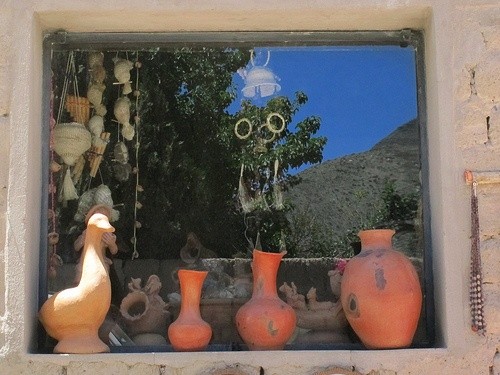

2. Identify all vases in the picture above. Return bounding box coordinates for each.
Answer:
[120,290,170,336]
[168,270,212,353]
[341,229,422,350]
[235,250,297,351]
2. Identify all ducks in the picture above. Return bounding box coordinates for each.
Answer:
[37,212,117,354]
